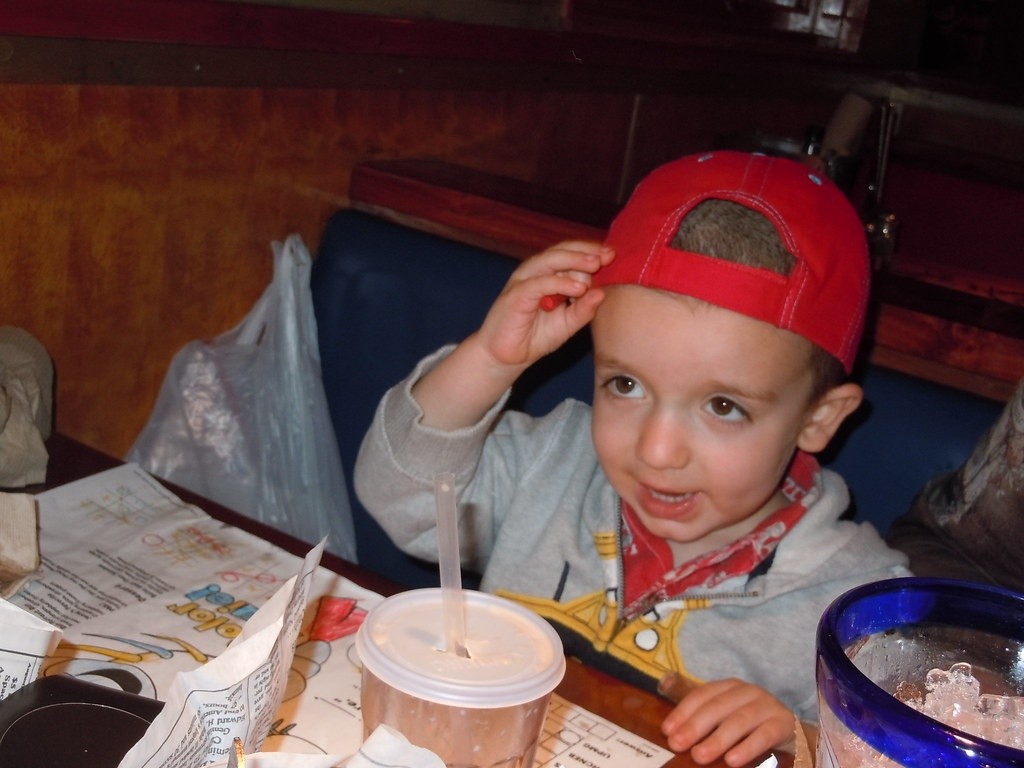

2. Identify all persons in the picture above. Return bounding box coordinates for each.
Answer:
[353,150,917,768]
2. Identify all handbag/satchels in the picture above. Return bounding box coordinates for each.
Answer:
[124,232,358,566]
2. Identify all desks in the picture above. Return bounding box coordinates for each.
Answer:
[845,157,1024,307]
[0,431,795,768]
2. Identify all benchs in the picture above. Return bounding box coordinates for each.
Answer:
[888,88,1024,185]
[310,154,1024,590]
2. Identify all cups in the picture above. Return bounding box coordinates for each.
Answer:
[353,588,567,768]
[813,571,1024,768]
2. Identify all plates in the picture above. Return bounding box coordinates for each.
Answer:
[0,673,166,768]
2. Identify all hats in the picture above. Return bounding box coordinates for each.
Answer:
[582,149,871,378]
